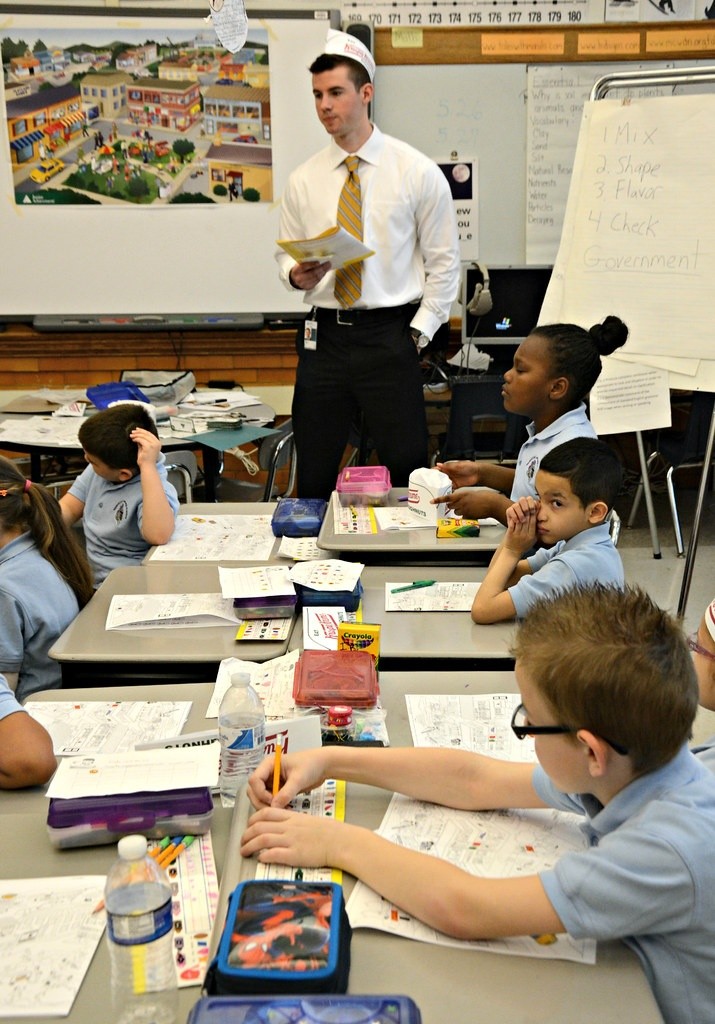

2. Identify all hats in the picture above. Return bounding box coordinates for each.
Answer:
[704,598,715,642]
[322,29,377,84]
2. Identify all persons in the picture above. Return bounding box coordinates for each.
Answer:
[244,579,715,1024]
[431,316,628,525]
[470,437,624,623]
[305,328,311,340]
[276,28,462,501]
[0,455,93,701]
[58,404,180,595]
[0,674,57,789]
[694,599,715,711]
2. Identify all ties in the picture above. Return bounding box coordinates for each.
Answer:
[334,156,365,310]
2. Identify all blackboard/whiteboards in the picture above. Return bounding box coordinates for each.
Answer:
[1,3,342,325]
[371,59,715,268]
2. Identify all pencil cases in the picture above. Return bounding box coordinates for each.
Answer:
[203,878,351,998]
[299,579,362,612]
[272,497,327,538]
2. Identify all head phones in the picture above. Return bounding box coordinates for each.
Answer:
[458,263,494,316]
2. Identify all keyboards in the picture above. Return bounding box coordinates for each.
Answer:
[449,374,509,386]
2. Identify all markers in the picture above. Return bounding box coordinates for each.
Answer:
[63,319,96,324]
[203,317,236,323]
[168,318,201,323]
[98,319,129,323]
[196,399,228,406]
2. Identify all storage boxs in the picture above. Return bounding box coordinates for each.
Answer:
[293,649,380,712]
[335,466,391,505]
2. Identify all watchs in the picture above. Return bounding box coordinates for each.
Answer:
[411,328,429,348]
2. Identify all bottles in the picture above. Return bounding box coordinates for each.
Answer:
[219,674,265,808]
[155,405,175,420]
[104,834,176,1024]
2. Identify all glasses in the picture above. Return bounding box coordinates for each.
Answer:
[687,632,715,661]
[512,704,631,756]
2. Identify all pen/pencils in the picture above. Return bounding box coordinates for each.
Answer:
[92,836,171,915]
[272,733,283,797]
[391,580,437,593]
[148,836,195,883]
[350,504,358,517]
[398,497,408,501]
[130,836,183,885]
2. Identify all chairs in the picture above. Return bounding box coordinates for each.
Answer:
[159,451,198,505]
[196,414,296,502]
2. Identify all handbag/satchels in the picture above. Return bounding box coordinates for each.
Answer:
[119,369,197,405]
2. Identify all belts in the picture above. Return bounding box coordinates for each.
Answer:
[311,304,422,325]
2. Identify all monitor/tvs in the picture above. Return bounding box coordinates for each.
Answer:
[463,263,554,346]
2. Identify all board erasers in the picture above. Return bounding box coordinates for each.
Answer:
[132,315,166,324]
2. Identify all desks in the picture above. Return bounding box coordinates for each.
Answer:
[0,386,666,1024]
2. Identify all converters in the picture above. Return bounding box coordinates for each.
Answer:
[208,380,235,389]
